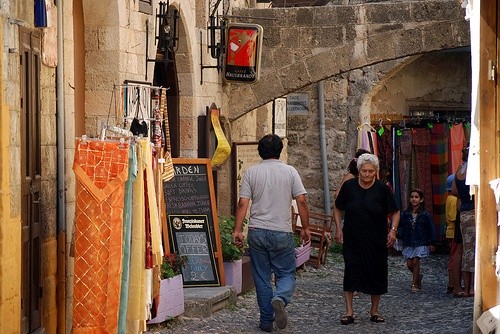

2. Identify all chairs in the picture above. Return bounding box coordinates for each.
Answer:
[292,205,334,269]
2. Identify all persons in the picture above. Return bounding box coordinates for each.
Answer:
[336,149,371,199]
[232,134,310,330]
[455,146,476,297]
[335,154,401,323]
[397,189,435,294]
[444,175,466,297]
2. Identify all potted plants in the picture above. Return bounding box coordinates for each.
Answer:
[146,251,189,324]
[216,213,248,295]
[292,233,311,267]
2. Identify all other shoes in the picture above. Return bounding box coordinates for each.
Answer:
[411,285,425,293]
[272,297,287,329]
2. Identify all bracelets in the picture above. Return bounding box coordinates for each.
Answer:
[391,226,398,231]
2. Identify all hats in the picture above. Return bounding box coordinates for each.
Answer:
[446,174,454,190]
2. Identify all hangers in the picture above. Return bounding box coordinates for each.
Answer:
[80,116,467,147]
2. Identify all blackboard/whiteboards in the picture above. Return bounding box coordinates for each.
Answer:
[161,158,226,288]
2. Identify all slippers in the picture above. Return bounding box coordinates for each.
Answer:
[370,315,385,323]
[341,315,354,325]
[453,291,464,298]
[447,286,453,294]
